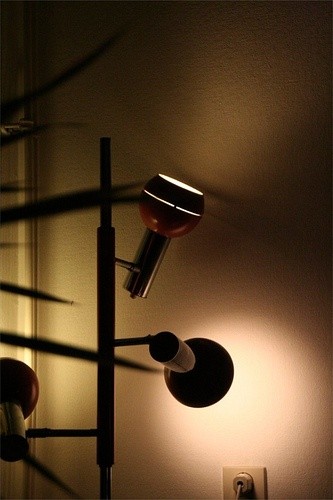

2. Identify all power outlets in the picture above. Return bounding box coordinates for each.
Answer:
[219,462,268,498]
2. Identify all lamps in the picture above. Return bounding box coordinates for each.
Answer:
[0,133,240,500]
